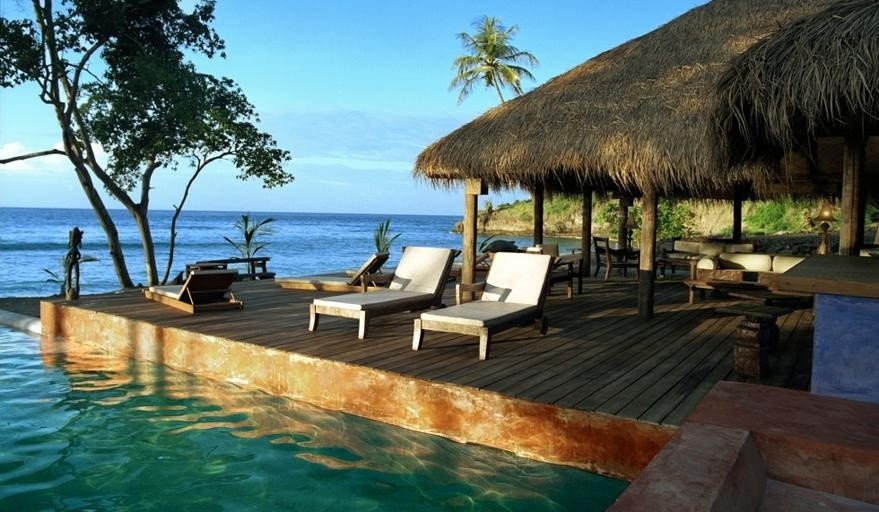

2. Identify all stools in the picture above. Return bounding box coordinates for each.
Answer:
[712,302,794,383]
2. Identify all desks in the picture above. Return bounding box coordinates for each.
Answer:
[773,252,879,402]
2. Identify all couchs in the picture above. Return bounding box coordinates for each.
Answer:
[688,252,814,299]
[658,236,756,278]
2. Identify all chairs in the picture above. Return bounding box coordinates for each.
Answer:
[277,250,389,294]
[306,246,458,340]
[592,237,638,280]
[537,243,583,299]
[141,269,245,315]
[409,251,556,359]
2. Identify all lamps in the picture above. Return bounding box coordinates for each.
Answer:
[813,194,835,257]
[618,210,645,247]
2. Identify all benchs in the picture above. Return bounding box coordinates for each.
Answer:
[182,257,270,279]
